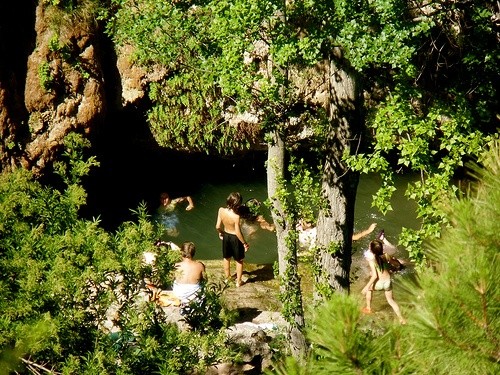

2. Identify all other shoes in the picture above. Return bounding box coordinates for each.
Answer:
[378,229,385,241]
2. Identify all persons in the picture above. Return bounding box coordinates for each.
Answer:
[85,239,276,375]
[361,240,407,326]
[216,192,249,287]
[157,193,194,238]
[295,217,319,252]
[236,197,276,239]
[376,228,398,251]
[353,222,378,240]
